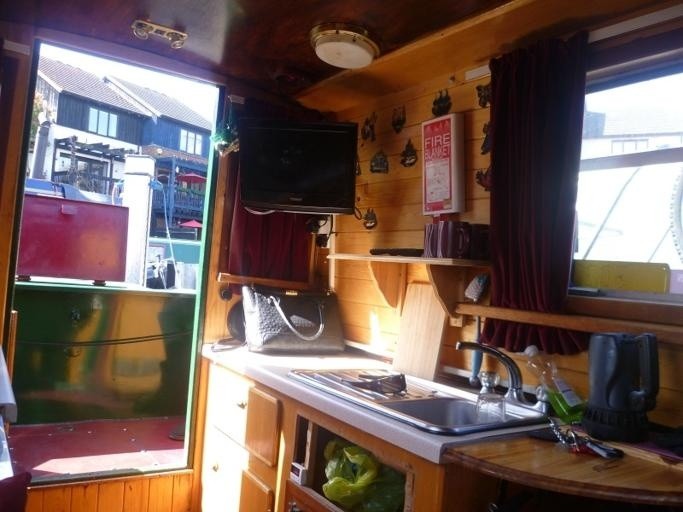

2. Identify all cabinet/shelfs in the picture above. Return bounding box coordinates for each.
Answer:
[284,405,584,511]
[200,364,283,511]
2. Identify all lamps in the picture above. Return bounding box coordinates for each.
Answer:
[306,21,386,70]
[129,17,188,50]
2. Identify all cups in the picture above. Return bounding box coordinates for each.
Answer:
[474,393,505,423]
[436,221,468,259]
[423,223,437,258]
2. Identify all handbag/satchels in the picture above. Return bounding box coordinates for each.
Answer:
[242,285,345,354]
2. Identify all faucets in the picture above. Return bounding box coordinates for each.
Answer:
[456,340,523,399]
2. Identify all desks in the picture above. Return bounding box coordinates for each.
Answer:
[443,434,682,511]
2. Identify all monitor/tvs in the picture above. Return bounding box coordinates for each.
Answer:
[236,116,357,214]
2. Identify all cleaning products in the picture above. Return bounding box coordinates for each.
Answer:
[521,344,587,427]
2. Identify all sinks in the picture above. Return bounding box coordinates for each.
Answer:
[379,389,552,438]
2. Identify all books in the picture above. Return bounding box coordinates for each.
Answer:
[422,218,491,260]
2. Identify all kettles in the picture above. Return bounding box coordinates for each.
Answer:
[581,333,660,443]
